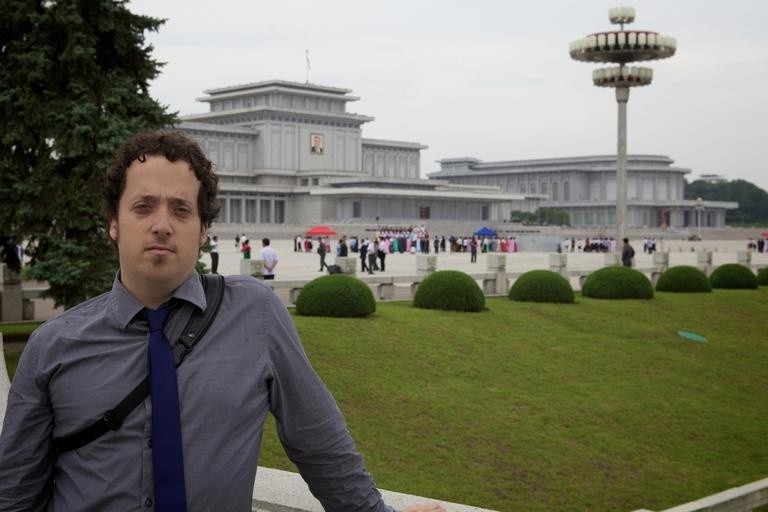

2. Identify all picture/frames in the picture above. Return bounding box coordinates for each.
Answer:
[309,133,324,156]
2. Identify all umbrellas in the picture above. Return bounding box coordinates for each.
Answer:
[304,224,338,238]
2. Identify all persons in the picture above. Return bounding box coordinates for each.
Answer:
[375,224,517,254]
[469,236,478,264]
[563,235,617,256]
[641,235,658,254]
[209,235,219,275]
[260,237,279,279]
[241,239,251,259]
[239,233,247,247]
[0,132,393,511]
[312,136,322,153]
[294,233,388,274]
[621,237,635,267]
[747,237,768,253]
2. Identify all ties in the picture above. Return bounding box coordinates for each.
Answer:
[144,306,191,510]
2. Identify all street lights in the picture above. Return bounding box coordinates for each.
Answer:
[568,4,676,265]
[694,198,705,239]
